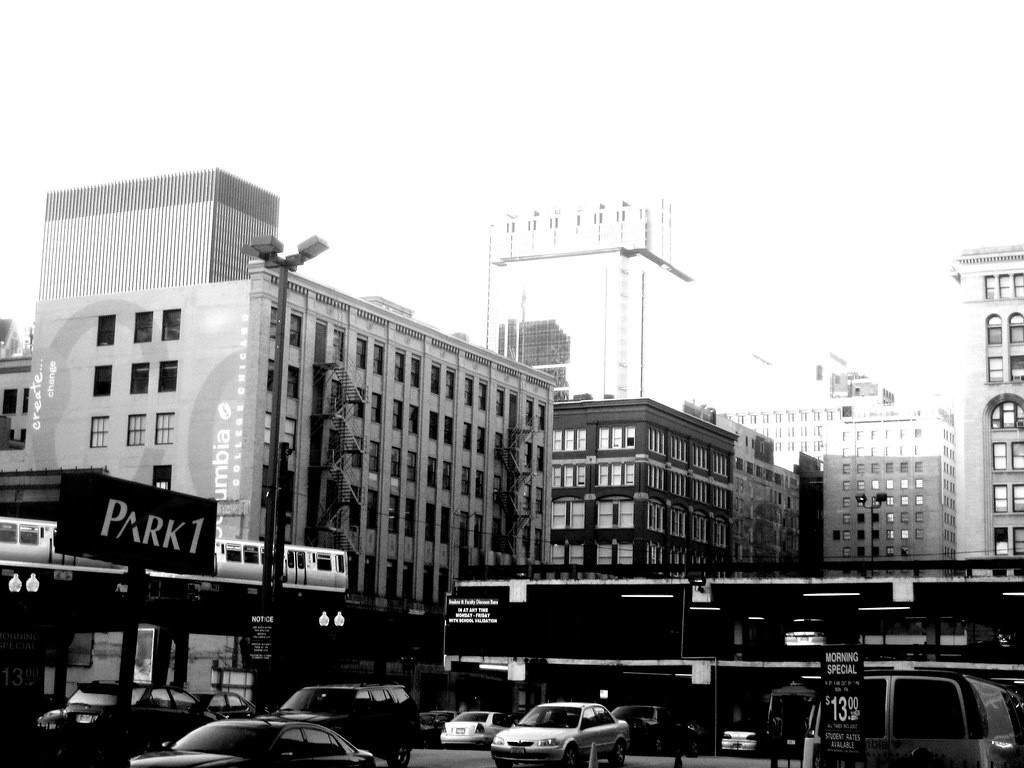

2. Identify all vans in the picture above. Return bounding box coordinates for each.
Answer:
[802,670,1024,768]
[272,683,419,768]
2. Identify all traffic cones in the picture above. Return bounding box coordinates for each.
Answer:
[587,741,599,768]
[673,750,682,768]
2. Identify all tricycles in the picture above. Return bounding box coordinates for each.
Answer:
[767,681,815,768]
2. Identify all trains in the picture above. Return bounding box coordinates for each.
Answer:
[0,513,350,594]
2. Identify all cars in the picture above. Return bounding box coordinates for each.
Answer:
[128,716,376,768]
[192,693,255,718]
[490,701,630,768]
[610,705,676,753]
[721,730,759,754]
[418,708,459,750]
[441,709,513,749]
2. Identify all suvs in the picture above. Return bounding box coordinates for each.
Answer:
[62,680,222,768]
